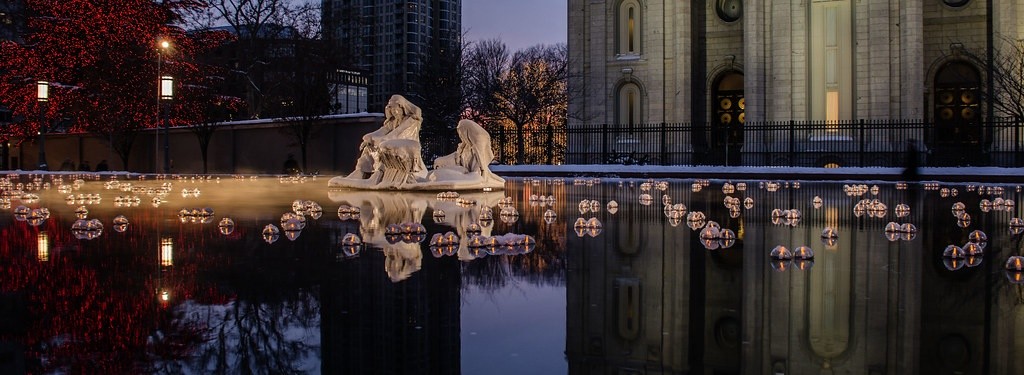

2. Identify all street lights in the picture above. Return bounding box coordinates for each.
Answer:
[155,208,173,301]
[156,41,173,172]
[36,80,48,172]
[37,222,49,264]
